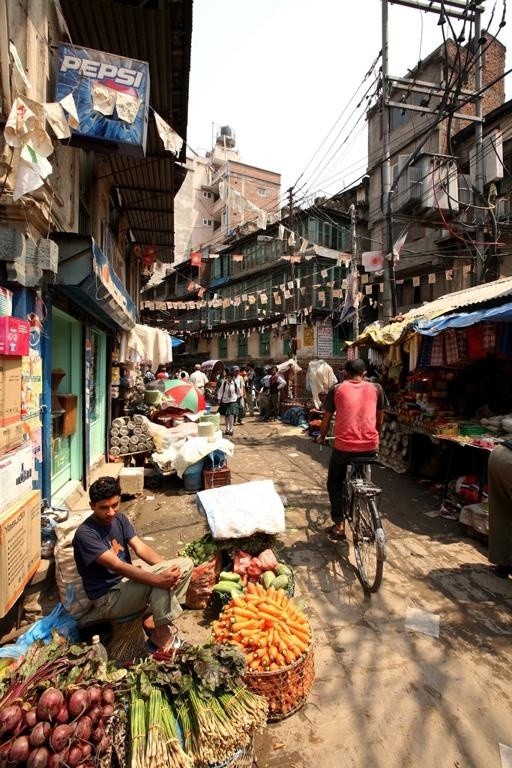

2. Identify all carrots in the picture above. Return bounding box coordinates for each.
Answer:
[209,580,315,716]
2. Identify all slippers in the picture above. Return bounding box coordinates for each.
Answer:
[325,524,346,540]
[144,634,192,653]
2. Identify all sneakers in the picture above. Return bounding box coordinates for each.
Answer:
[229,429,233,434]
[226,429,229,434]
[238,418,245,424]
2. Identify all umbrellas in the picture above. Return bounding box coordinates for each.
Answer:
[165,381,207,411]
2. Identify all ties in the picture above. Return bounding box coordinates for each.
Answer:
[228,384,231,398]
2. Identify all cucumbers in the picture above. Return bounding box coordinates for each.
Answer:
[212,571,244,595]
[260,562,293,592]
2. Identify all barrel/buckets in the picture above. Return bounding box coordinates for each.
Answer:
[184,462,203,493]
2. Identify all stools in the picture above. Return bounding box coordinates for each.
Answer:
[104,611,147,665]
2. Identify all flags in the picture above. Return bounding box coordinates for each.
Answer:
[3,81,353,263]
[139,264,467,339]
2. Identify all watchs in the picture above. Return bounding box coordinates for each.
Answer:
[189,362,210,395]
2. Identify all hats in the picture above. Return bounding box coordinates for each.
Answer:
[231,365,240,371]
[195,363,201,369]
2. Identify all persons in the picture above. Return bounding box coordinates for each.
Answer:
[177,366,189,380]
[155,367,168,379]
[70,472,198,659]
[141,369,156,384]
[486,438,511,579]
[179,372,189,383]
[256,366,273,416]
[268,364,288,420]
[315,357,391,541]
[214,359,257,435]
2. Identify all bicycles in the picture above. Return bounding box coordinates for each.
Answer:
[314,432,387,593]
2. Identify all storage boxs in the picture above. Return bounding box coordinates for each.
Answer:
[202,462,231,490]
[118,467,144,496]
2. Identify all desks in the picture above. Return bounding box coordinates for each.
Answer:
[397,418,512,503]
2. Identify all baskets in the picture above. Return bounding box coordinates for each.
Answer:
[241,619,315,720]
[101,706,254,767]
